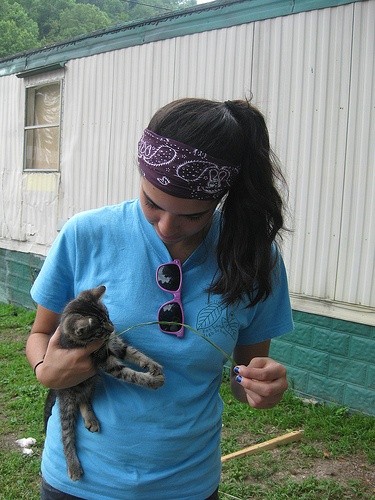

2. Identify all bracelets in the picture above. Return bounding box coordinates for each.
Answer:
[33,361,43,375]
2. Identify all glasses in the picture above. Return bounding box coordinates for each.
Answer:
[154,258,186,338]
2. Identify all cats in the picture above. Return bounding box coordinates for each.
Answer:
[42,286,164,484]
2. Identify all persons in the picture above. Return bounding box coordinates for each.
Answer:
[26,99,294,500]
